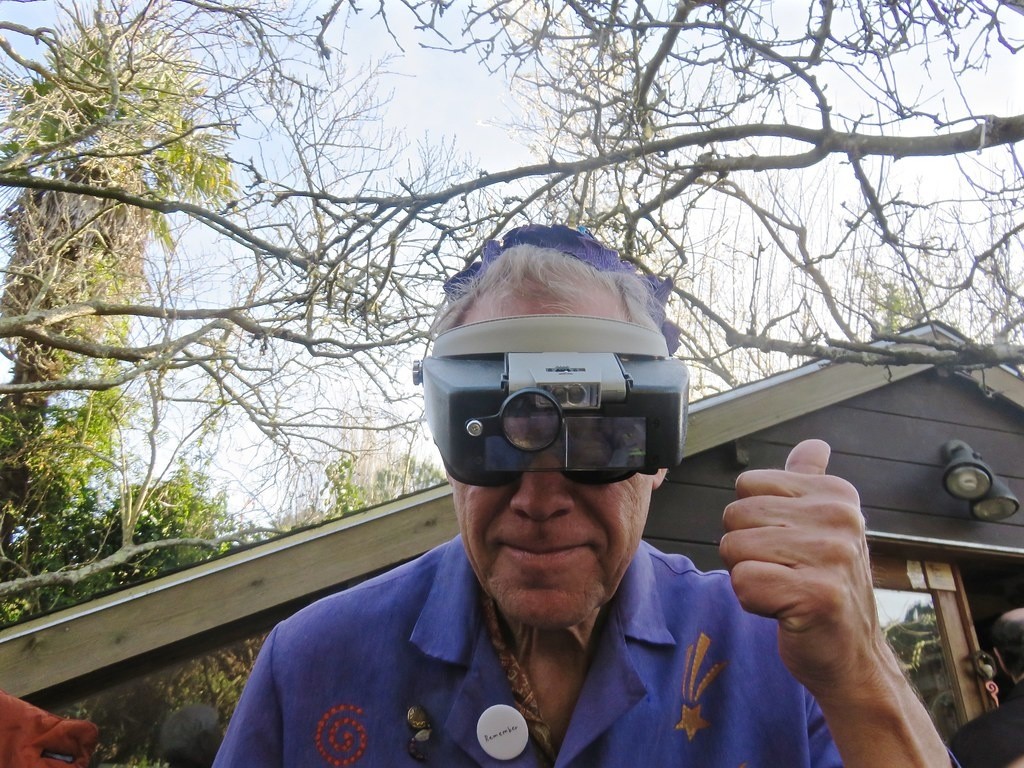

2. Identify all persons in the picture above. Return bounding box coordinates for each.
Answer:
[949,607,1024,768]
[213,224,963,768]
[160,702,225,768]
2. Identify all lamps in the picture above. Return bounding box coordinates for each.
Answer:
[942,439,1019,521]
